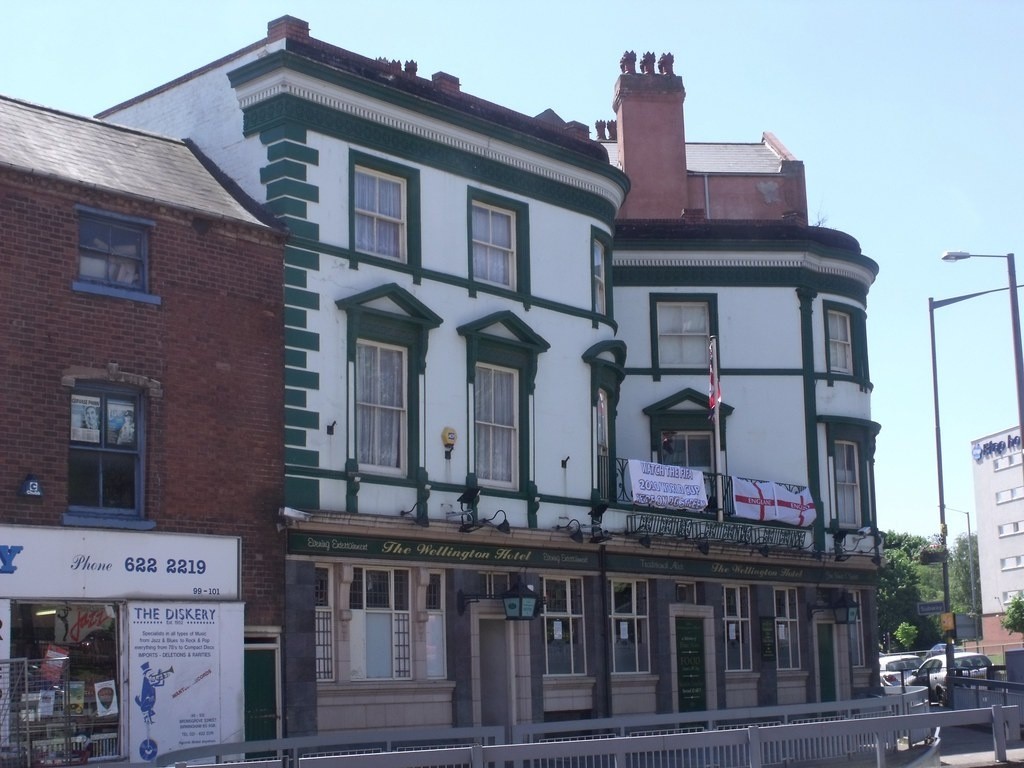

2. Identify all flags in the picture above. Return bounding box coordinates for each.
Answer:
[707,343,722,423]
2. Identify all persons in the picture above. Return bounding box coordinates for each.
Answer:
[81,406,100,429]
[116,409,135,444]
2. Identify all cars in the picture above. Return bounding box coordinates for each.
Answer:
[926,644,962,658]
[906,652,993,707]
[879,654,923,687]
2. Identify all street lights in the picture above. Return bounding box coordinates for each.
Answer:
[941,251,1024,490]
[927,284,1024,709]
